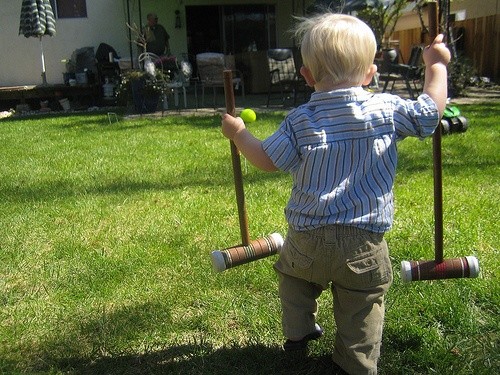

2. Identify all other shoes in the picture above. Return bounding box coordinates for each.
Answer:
[284,323,322,351]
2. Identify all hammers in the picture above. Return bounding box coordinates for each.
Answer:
[209,67,284,274]
[397,0,480,283]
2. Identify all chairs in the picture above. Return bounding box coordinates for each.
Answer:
[196,51,246,108]
[260,47,308,108]
[138,51,192,110]
[381,45,424,98]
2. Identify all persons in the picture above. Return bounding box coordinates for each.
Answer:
[137,12,170,63]
[221,0,451,375]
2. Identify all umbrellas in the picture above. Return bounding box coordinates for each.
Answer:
[18,0,57,84]
